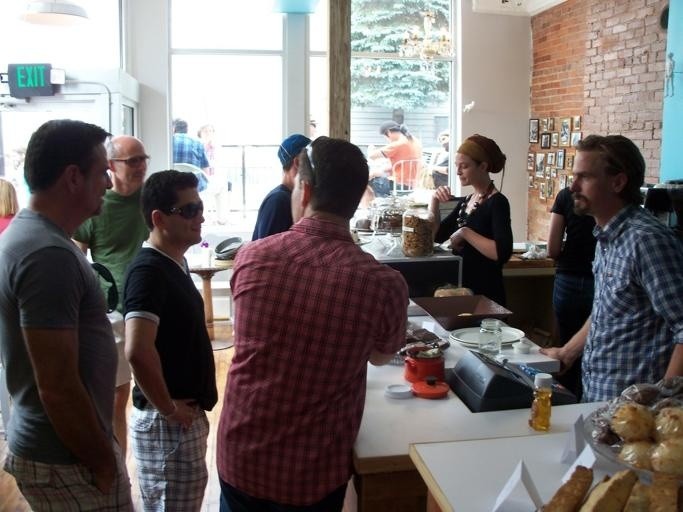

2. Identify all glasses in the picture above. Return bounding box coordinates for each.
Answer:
[170,201,202,218]
[306,136,328,185]
[113,155,150,167]
[92,262,118,313]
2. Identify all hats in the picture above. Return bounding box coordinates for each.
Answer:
[278,134,311,165]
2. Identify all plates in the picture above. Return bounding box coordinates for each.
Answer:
[450,325,522,346]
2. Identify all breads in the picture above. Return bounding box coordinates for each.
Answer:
[532,464,683,512]
[594,376,683,476]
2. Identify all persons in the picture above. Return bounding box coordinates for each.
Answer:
[431,134,448,188]
[252,134,313,243]
[429,133,512,327]
[70,137,158,462]
[547,186,596,402]
[217,136,410,511]
[393,107,405,126]
[538,130,682,402]
[362,121,421,208]
[0,179,19,234]
[150,119,210,194]
[125,168,218,512]
[0,119,133,511]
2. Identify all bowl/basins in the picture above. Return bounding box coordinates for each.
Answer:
[580,409,682,485]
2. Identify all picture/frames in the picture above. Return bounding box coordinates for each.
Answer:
[527,116,583,203]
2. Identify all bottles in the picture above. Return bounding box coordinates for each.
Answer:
[531,370,551,433]
[482,318,503,362]
[401,203,436,257]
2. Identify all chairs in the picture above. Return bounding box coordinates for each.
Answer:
[171,162,209,195]
[390,158,423,194]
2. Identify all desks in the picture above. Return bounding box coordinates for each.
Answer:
[185,254,236,349]
[408,431,648,512]
[352,315,614,511]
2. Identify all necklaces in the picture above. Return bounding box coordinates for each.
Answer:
[456,180,494,228]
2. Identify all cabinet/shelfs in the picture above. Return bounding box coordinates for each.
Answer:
[351,232,462,298]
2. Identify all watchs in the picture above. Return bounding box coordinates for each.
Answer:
[158,399,178,419]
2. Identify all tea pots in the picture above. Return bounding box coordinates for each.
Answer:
[397,340,451,382]
